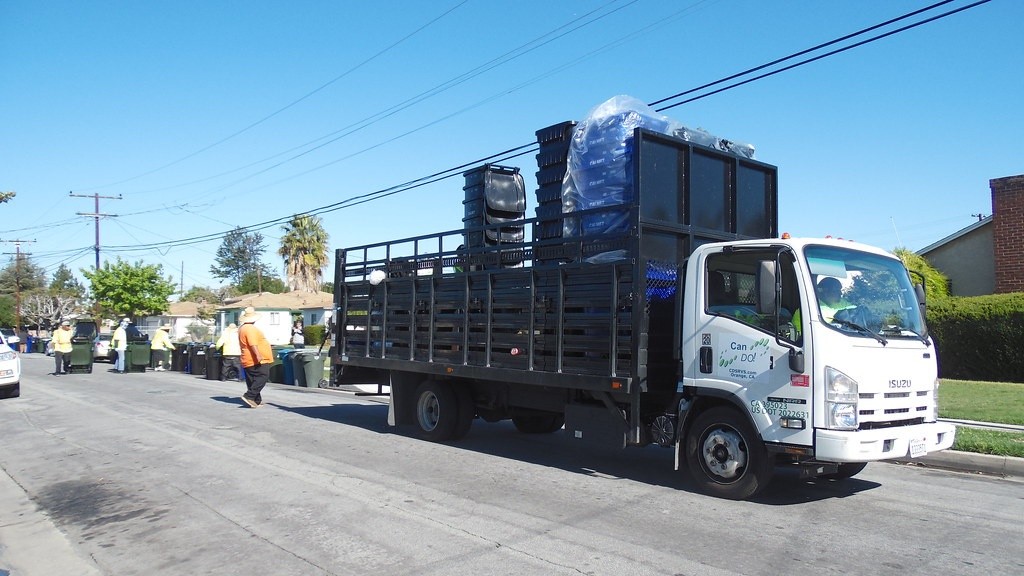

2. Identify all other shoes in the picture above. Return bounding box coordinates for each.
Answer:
[257,401,266,407]
[155,366,165,372]
[242,396,257,407]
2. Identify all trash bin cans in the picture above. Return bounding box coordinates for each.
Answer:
[68,336,93,373]
[2,335,55,354]
[461,163,525,276]
[118,340,152,374]
[278,350,326,389]
[536,110,755,265]
[171,341,243,380]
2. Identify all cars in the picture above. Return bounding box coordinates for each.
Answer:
[92,333,115,362]
[0,332,22,398]
[0,328,20,353]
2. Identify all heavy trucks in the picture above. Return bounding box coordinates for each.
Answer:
[324,94,957,502]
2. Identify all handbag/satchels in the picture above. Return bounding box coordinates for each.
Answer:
[834,306,882,334]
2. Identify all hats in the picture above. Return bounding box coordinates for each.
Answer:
[159,324,173,329]
[239,307,262,323]
[121,318,133,324]
[62,321,69,326]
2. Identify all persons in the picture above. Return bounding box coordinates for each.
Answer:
[792,277,857,335]
[10,327,16,350]
[111,318,133,374]
[291,321,305,349]
[238,306,274,408]
[215,323,243,382]
[151,324,176,372]
[52,321,73,377]
[18,327,29,353]
